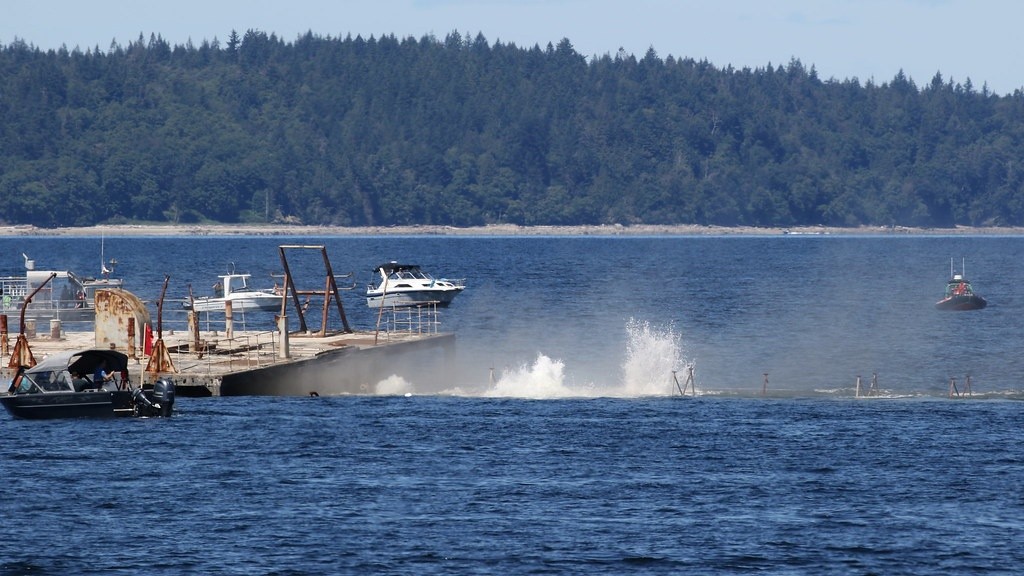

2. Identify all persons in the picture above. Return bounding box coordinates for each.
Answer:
[68,359,115,392]
[44,284,87,308]
[272,283,283,296]
[951,281,967,295]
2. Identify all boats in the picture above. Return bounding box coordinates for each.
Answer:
[366,262,468,310]
[182,262,293,314]
[0,231,148,323]
[0,349,174,419]
[934,259,986,312]
